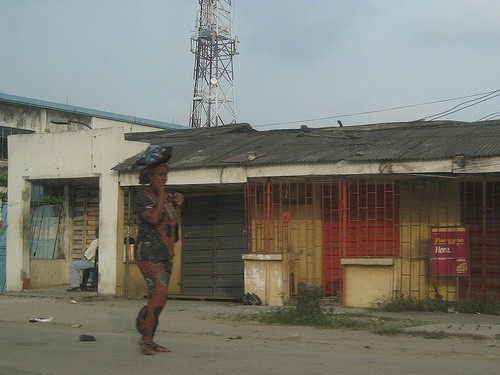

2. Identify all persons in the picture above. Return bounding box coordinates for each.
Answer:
[132,163,185,355]
[67,224,99,293]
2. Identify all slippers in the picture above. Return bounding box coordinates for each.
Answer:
[138,337,170,355]
[66,287,82,292]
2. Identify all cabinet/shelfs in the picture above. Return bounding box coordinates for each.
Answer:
[341,258,398,308]
[242,253,299,306]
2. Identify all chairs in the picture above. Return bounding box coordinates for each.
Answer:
[80,246,98,292]
